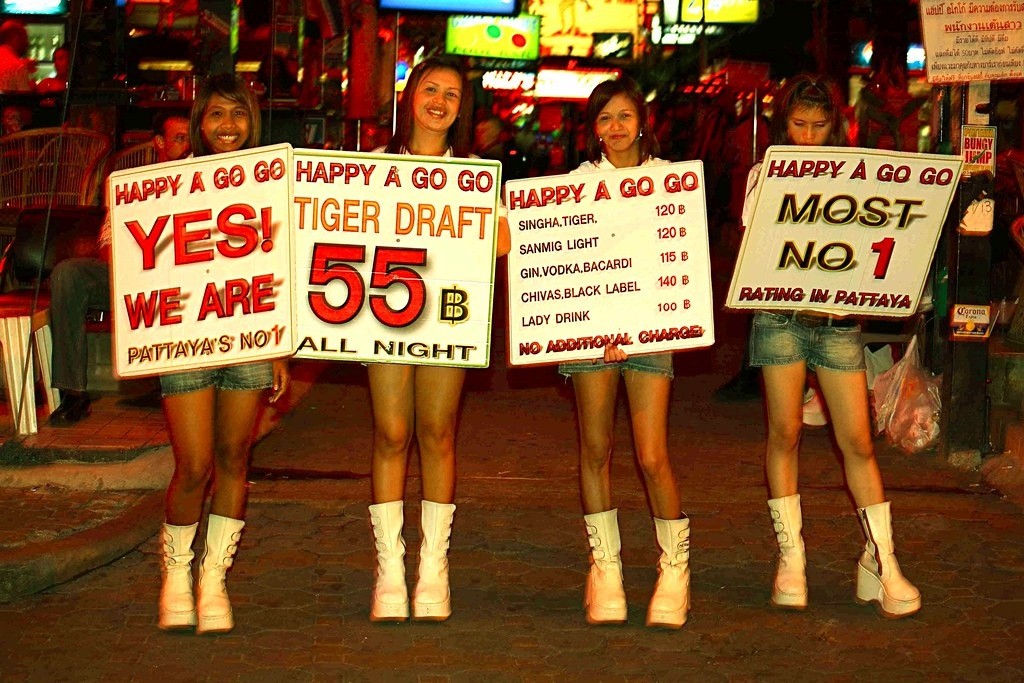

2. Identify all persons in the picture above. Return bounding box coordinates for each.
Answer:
[471,104,586,182]
[360,59,511,623]
[741,75,922,618]
[559,80,692,631]
[656,85,771,260]
[49,116,193,426]
[0,19,71,94]
[158,73,289,634]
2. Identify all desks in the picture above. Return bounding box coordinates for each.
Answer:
[0,84,197,151]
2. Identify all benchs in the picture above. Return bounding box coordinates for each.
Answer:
[0,204,164,395]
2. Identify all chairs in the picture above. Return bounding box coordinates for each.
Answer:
[0,127,159,254]
[861,316,921,363]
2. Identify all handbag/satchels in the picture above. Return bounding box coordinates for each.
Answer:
[874,336,942,456]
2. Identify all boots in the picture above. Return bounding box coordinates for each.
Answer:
[857,501,921,617]
[582,507,628,624]
[159,518,198,630]
[767,494,808,611]
[198,514,245,632]
[646,510,690,629]
[366,500,409,623]
[415,500,457,621]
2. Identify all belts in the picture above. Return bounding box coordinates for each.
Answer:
[773,311,860,329]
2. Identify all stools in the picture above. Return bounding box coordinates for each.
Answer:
[0,304,63,435]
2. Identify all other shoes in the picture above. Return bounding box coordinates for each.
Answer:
[50,392,88,427]
